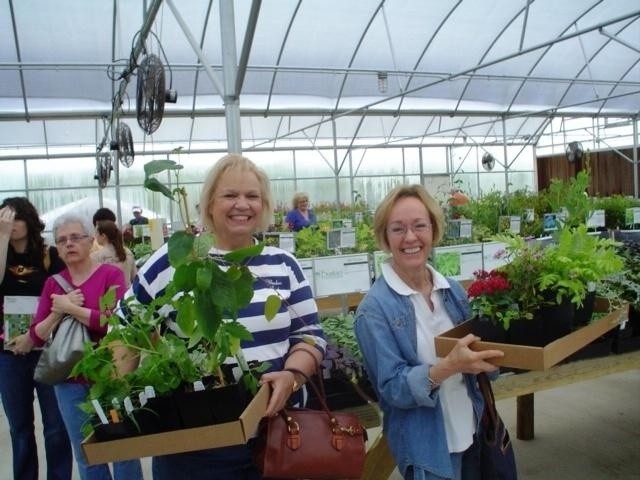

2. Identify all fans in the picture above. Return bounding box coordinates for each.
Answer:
[480,151,496,172]
[91,33,180,191]
[566,141,584,164]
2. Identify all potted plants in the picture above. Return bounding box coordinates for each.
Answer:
[533,220,627,327]
[66,224,317,440]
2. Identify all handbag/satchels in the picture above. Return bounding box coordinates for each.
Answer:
[259,348,368,480]
[32,274,95,388]
[476,371,518,480]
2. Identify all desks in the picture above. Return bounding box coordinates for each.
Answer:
[307,347,640,443]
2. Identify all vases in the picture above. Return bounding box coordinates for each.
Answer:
[474,305,570,346]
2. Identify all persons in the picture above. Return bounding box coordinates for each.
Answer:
[284,191,316,231]
[352,183,519,479]
[29,210,145,480]
[103,153,328,480]
[128,205,148,224]
[448,185,468,217]
[90,208,117,251]
[0,196,72,480]
[91,219,137,290]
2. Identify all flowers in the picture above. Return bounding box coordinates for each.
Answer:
[466,229,556,332]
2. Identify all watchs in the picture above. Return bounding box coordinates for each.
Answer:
[289,373,304,394]
